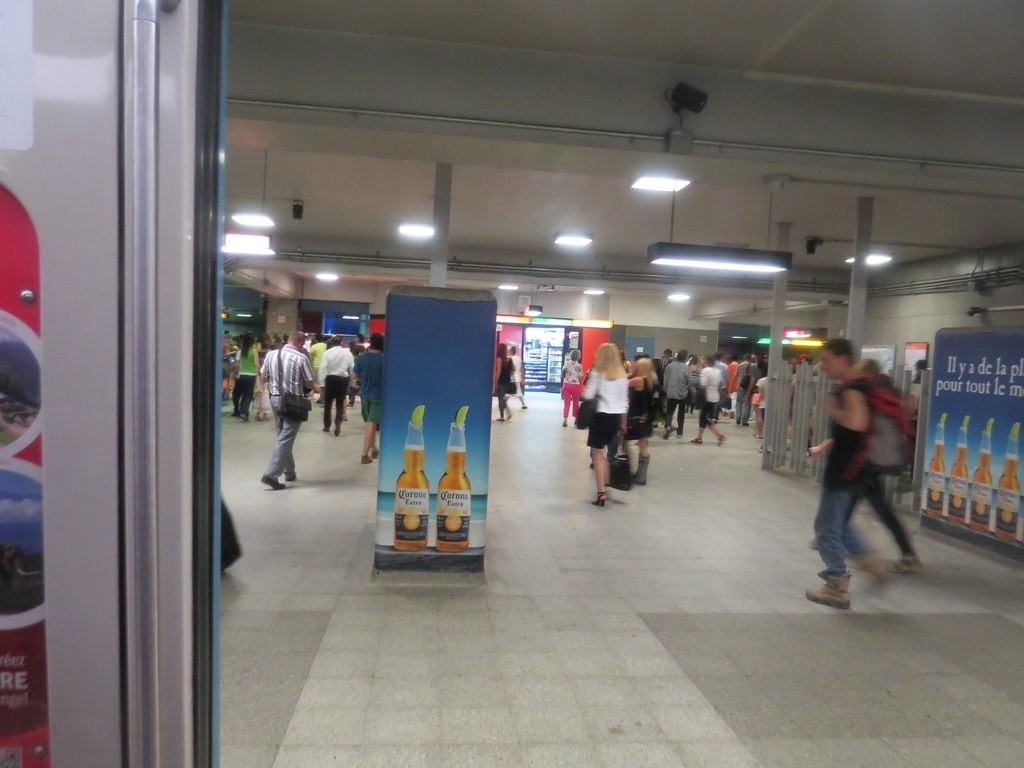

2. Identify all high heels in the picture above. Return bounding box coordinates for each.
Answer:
[592,491,605,507]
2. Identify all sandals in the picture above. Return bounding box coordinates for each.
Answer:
[717,435,725,446]
[690,438,703,444]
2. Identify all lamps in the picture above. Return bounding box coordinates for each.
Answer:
[647,191,791,274]
[523,288,543,317]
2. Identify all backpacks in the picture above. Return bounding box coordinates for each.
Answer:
[849,385,915,475]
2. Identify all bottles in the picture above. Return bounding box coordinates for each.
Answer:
[993,434,1020,540]
[948,427,969,524]
[969,431,992,532]
[394,420,431,551]
[436,422,472,552]
[927,423,945,515]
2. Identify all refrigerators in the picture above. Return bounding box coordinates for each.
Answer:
[546,346,563,392]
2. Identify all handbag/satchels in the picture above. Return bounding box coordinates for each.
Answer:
[507,382,517,394]
[624,415,651,441]
[740,374,757,392]
[576,398,597,429]
[722,394,731,410]
[610,436,631,490]
[276,394,311,422]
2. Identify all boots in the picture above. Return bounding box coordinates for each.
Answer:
[632,453,650,485]
[805,575,851,608]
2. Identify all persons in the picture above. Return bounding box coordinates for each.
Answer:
[802,337,922,609]
[626,358,655,484]
[352,333,384,464]
[560,350,582,427]
[223,330,385,436]
[493,343,528,421]
[582,343,629,507]
[588,350,810,469]
[261,331,314,489]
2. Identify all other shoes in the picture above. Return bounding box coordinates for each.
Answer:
[885,558,920,573]
[742,423,749,426]
[497,417,504,421]
[261,475,285,490]
[663,428,673,439]
[284,470,296,481]
[322,426,330,431]
[370,450,381,460]
[362,454,373,464]
[334,421,339,436]
[563,417,567,426]
[230,411,269,424]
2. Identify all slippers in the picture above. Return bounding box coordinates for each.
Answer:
[754,433,762,438]
